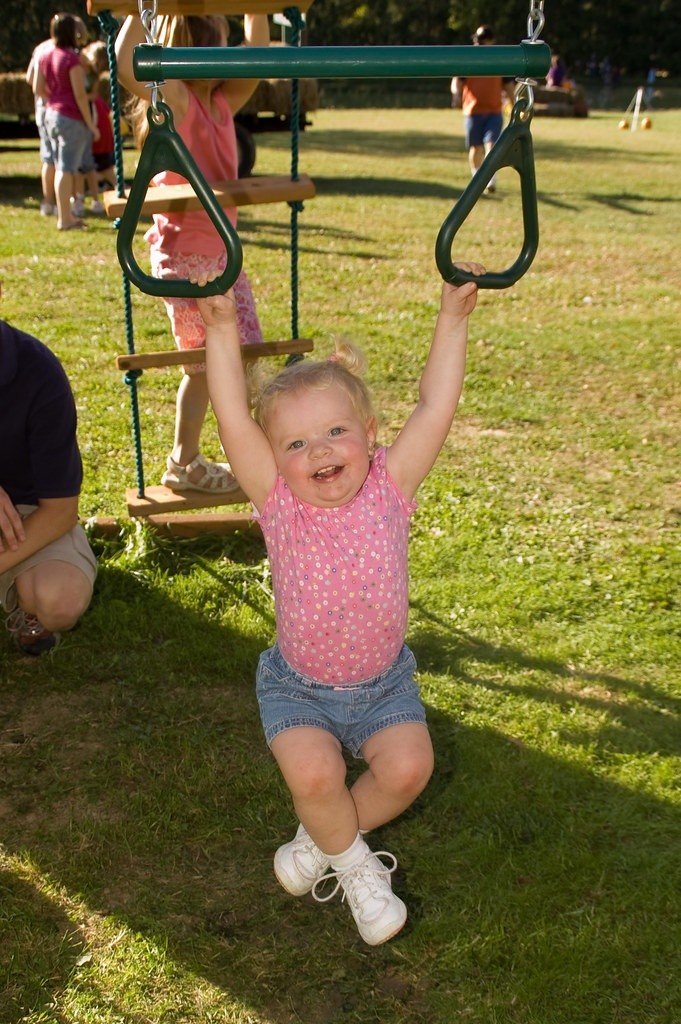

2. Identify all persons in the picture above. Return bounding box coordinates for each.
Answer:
[25,14,122,228]
[186,261,488,947]
[451,26,519,192]
[115,9,271,494]
[0,323,99,655]
[546,51,565,87]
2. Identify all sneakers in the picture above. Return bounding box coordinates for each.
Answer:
[273,823,331,897]
[311,844,407,946]
[3,606,55,657]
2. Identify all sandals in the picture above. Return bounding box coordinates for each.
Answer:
[160,452,240,493]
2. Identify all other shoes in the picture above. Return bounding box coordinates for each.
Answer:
[58,217,89,232]
[90,204,104,213]
[71,201,85,216]
[483,186,496,197]
[39,200,58,216]
